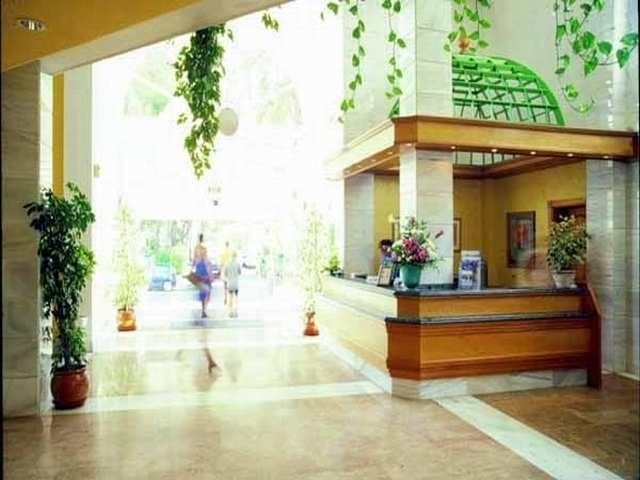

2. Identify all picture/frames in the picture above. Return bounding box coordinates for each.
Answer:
[506,211,536,268]
[547,198,586,229]
[391,216,462,252]
[377,263,396,287]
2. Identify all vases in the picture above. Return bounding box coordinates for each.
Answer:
[399,263,424,292]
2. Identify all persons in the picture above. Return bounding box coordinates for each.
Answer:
[191,233,212,318]
[221,251,241,317]
[219,242,232,304]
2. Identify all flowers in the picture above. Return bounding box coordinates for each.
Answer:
[379,216,444,278]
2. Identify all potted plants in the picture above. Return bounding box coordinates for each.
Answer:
[325,224,341,276]
[21,182,94,409]
[544,215,591,288]
[111,198,147,330]
[295,209,325,336]
